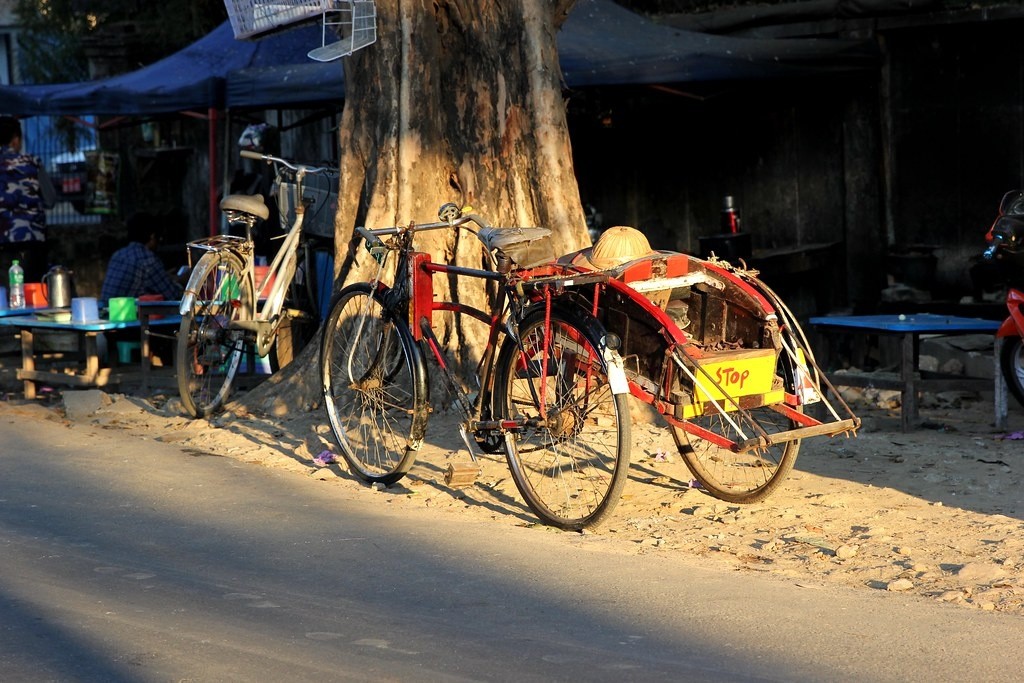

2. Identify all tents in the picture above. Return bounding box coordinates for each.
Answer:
[0,1,896,286]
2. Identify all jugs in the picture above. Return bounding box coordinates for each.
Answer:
[40,265,73,310]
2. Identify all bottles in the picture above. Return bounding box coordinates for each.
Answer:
[8,260,26,311]
[723,196,741,234]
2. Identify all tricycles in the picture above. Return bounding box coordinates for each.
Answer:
[318,203,862,532]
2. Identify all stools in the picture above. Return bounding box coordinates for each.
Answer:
[117,342,140,363]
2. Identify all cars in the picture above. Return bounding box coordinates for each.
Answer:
[48,145,97,216]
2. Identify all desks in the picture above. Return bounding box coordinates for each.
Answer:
[811,314,1006,435]
[732,244,833,280]
[0,299,284,398]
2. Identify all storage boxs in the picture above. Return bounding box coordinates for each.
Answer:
[223,0,337,42]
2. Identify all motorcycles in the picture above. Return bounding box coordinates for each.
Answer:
[983,189,1024,409]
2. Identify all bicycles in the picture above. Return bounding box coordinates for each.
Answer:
[176,150,341,417]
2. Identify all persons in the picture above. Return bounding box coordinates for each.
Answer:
[102,215,190,365]
[0,116,46,285]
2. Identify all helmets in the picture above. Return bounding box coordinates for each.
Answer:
[576,226,660,271]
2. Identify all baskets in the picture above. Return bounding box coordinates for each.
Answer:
[280,165,339,238]
